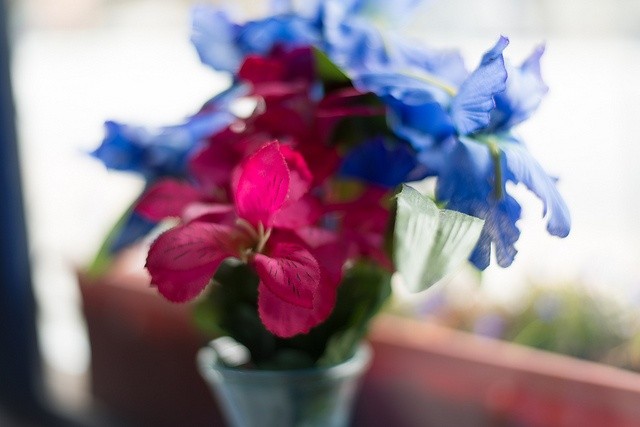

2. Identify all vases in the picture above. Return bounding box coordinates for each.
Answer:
[194,338,376,427]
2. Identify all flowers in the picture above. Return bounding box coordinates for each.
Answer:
[75,1,571,372]
